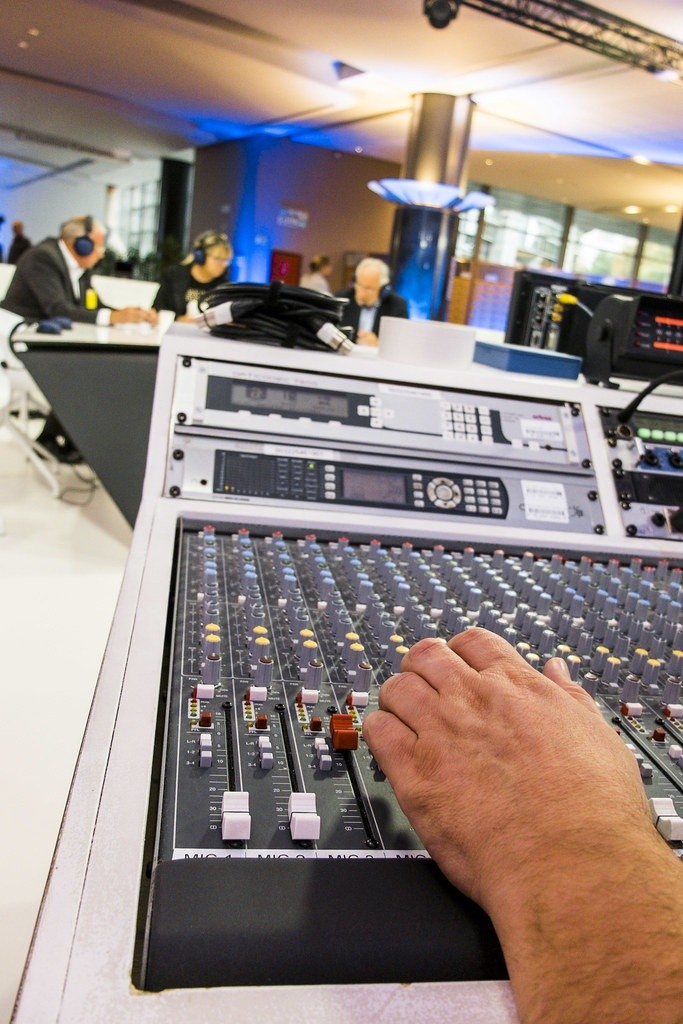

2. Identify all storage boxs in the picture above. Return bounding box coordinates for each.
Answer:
[475,341,584,382]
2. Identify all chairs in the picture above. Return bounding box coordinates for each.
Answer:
[1,308,52,426]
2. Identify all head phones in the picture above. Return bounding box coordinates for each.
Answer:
[193,234,221,265]
[9,315,72,352]
[352,275,393,300]
[74,217,93,257]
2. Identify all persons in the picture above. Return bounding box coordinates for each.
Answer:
[8,219,32,264]
[152,231,234,322]
[332,257,408,347]
[0,215,156,463]
[364,631,683,1024]
[300,256,332,294]
